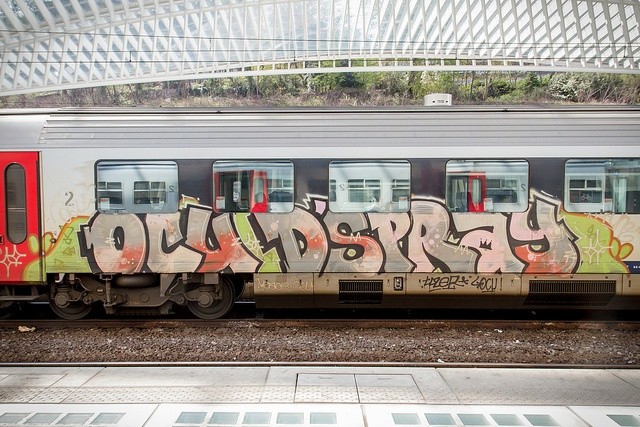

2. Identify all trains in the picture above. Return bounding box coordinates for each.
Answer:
[1,100,637,322]
